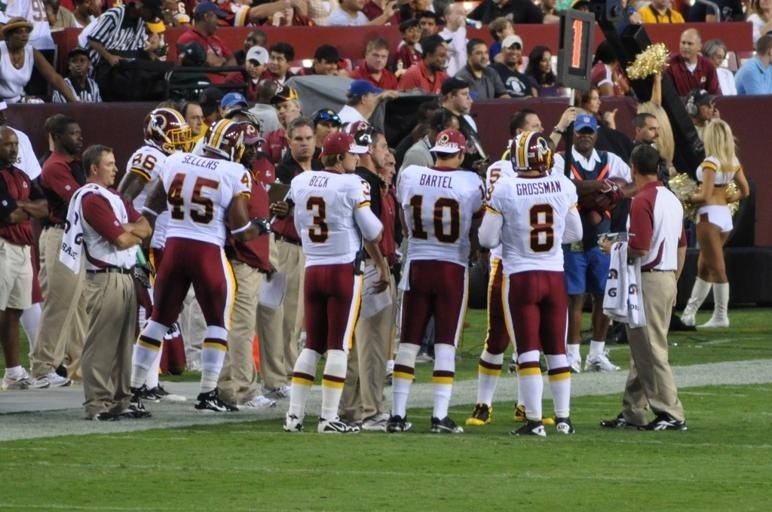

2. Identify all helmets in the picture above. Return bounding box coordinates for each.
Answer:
[143,107,195,153]
[203,118,245,162]
[511,131,554,171]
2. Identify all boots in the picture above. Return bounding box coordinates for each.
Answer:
[698,283,730,327]
[681,276,713,326]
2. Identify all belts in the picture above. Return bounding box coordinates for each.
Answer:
[275,233,301,245]
[86,267,130,274]
[45,222,64,228]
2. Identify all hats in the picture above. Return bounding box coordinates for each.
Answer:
[428,129,465,153]
[423,35,452,49]
[442,77,470,94]
[573,115,598,132]
[351,80,381,94]
[141,6,166,34]
[502,35,523,48]
[176,41,206,64]
[246,45,269,65]
[269,85,297,102]
[221,92,248,108]
[316,45,342,62]
[314,109,341,125]
[241,121,267,144]
[689,88,718,103]
[195,2,227,19]
[2,16,33,32]
[322,132,369,154]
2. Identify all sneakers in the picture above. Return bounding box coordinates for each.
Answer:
[601,413,647,428]
[514,403,554,424]
[567,350,580,372]
[431,416,464,433]
[238,395,275,409]
[194,395,239,413]
[361,413,411,431]
[284,412,303,432]
[124,401,151,418]
[130,386,159,402]
[637,412,689,431]
[2,374,49,390]
[94,410,119,422]
[511,419,546,436]
[466,403,492,425]
[556,417,575,434]
[584,352,620,371]
[151,386,171,400]
[37,372,71,387]
[387,414,406,433]
[317,417,359,433]
[263,385,290,398]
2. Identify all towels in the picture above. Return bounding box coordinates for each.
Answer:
[601,239,648,331]
[58,182,128,275]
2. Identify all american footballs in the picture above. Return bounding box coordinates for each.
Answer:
[597,178,627,210]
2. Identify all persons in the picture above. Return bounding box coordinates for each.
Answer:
[1,0,770,440]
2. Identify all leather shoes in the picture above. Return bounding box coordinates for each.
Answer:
[616,327,626,344]
[667,317,695,331]
